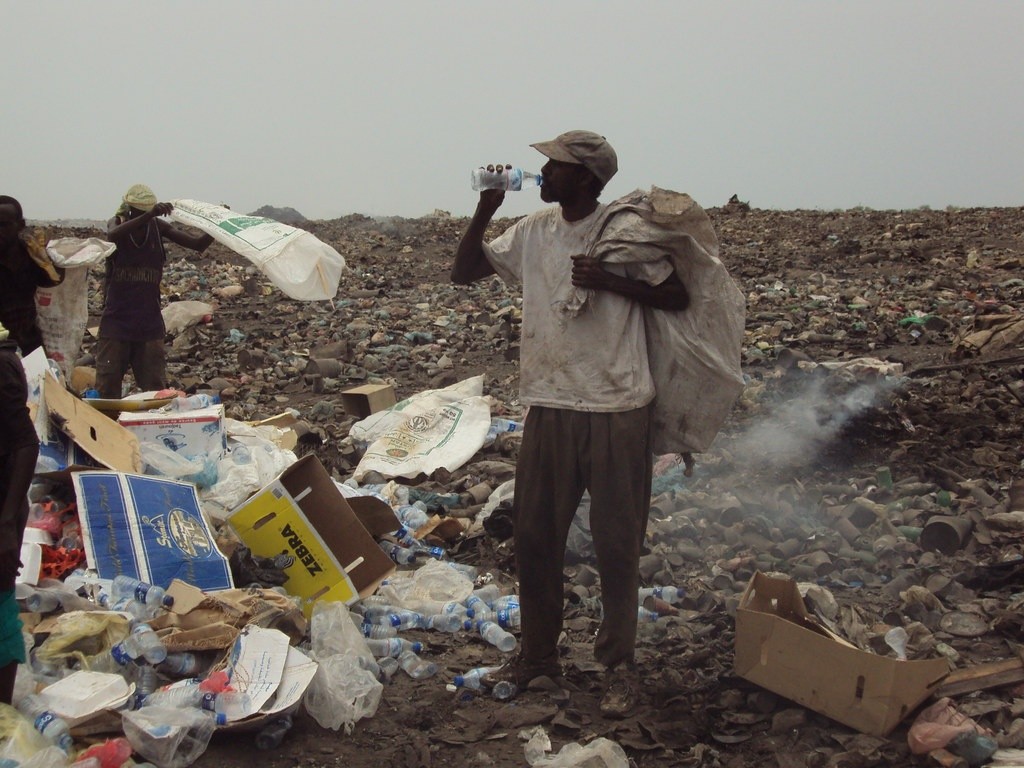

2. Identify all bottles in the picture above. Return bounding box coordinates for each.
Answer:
[377,500,449,565]
[360,593,521,700]
[17,697,73,752]
[472,167,542,192]
[496,418,522,435]
[24,586,70,614]
[170,394,221,411]
[637,585,688,622]
[253,715,292,748]
[96,575,251,730]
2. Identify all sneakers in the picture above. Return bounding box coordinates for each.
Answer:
[600,665,639,716]
[479,651,562,688]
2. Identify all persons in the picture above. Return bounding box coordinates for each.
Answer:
[0,322,40,707]
[449,129,691,718]
[0,194,66,357]
[93,183,231,420]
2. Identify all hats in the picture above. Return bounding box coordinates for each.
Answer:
[528,130,618,186]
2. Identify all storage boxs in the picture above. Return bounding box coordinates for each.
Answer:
[730,569,953,738]
[230,452,399,621]
